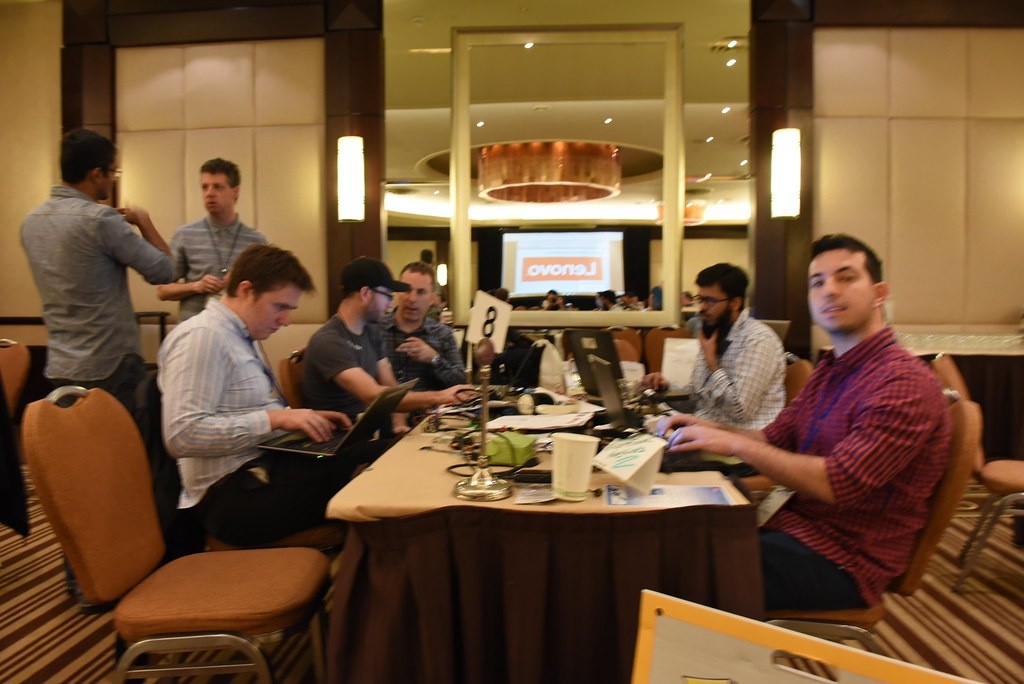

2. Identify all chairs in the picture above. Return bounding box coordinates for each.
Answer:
[0,327,1024,684]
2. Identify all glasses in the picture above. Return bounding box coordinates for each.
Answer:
[690,295,730,307]
[369,288,395,301]
[107,167,123,180]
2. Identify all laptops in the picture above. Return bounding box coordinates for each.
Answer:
[469,342,548,402]
[587,353,744,473]
[562,327,690,404]
[254,377,419,456]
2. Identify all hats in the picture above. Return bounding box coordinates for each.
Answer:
[342,258,411,292]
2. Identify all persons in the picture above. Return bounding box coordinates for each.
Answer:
[380,262,467,436]
[153,156,270,326]
[639,262,787,480]
[540,290,565,311]
[652,234,952,619]
[594,286,693,312]
[419,249,442,299]
[156,244,362,553]
[301,255,477,452]
[20,128,166,614]
[486,289,510,303]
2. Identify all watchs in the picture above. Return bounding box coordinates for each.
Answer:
[430,353,441,365]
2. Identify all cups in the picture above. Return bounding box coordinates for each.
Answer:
[551,431,601,504]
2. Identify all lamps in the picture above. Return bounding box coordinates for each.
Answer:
[478,141,622,204]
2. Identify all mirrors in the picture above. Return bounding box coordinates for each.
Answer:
[382,0,759,361]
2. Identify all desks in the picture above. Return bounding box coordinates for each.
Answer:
[327,404,761,684]
